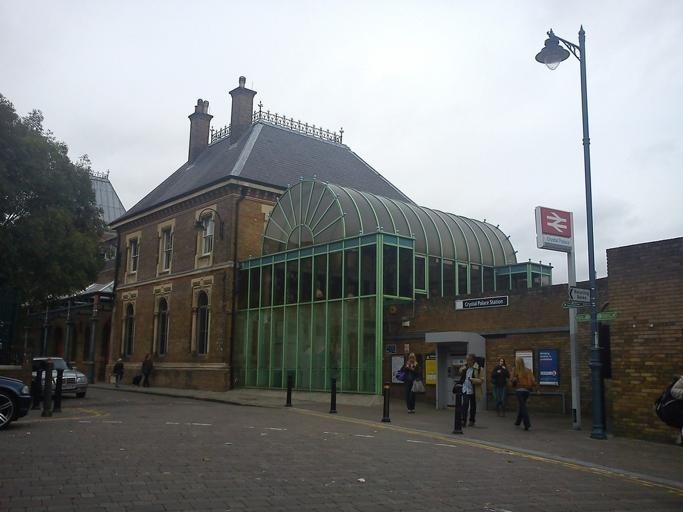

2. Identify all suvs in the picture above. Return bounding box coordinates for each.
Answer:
[32,358,89,399]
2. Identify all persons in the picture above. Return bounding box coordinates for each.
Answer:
[141,352,153,387]
[510,358,539,431]
[459,354,485,427]
[491,358,510,418]
[671,376,683,400]
[400,352,420,414]
[112,357,124,387]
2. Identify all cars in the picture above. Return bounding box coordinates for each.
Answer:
[0,376,34,430]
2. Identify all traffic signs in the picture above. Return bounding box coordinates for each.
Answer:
[561,303,585,309]
[575,312,617,321]
[567,287,591,303]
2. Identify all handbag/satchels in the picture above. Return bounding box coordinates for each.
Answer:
[654,376,682,428]
[395,365,405,381]
[511,367,517,386]
[410,378,425,393]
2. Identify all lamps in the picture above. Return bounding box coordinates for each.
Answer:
[193,208,223,239]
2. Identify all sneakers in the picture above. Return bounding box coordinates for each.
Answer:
[468,420,473,426]
[461,420,466,426]
[524,423,530,431]
[407,409,411,413]
[411,409,415,412]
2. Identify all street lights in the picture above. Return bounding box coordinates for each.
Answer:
[535,27,606,432]
